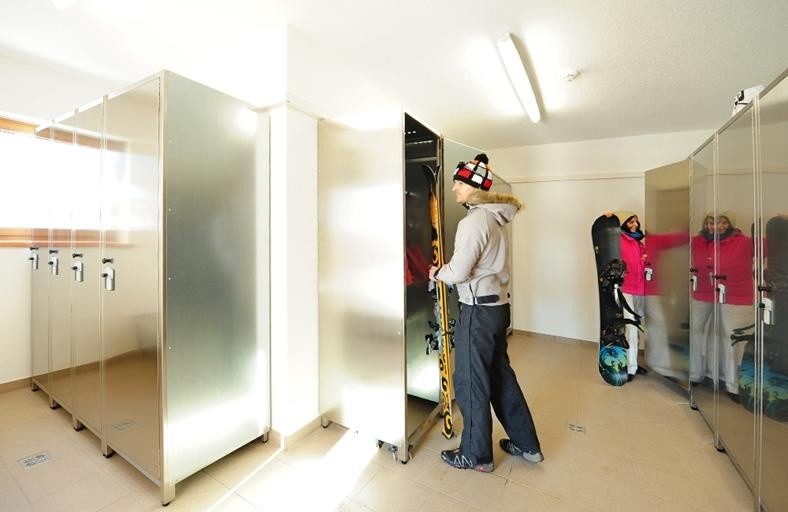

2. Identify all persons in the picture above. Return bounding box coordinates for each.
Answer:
[643,229,690,385]
[426,153,545,473]
[689,211,714,387]
[706,209,787,406]
[601,207,648,384]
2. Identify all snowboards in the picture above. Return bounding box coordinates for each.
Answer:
[591,214,643,386]
[730,215,788,424]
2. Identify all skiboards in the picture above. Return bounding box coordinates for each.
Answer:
[422,163,457,439]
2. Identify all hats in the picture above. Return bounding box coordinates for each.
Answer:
[453,153,493,191]
[616,208,637,226]
[721,210,736,229]
[704,212,721,220]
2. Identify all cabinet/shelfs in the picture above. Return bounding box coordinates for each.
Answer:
[27,71,272,509]
[643,68,788,512]
[316,112,514,466]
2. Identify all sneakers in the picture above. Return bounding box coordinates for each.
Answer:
[441,449,494,472]
[500,438,544,463]
[628,366,648,381]
[729,391,740,404]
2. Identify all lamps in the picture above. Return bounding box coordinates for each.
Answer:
[494,32,541,123]
